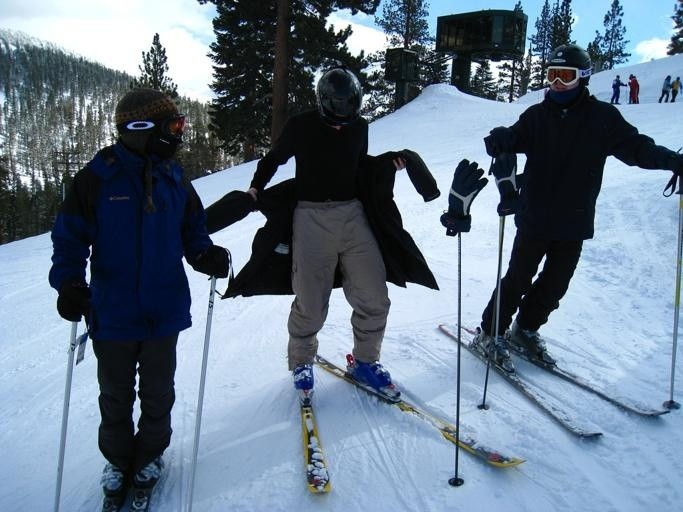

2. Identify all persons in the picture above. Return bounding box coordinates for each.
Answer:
[473,42,683,362]
[48,88,230,497]
[609,73,683,105]
[203,71,439,389]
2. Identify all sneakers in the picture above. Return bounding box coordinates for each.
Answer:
[510,320,548,357]
[100,460,130,497]
[131,454,165,490]
[476,329,511,367]
[352,357,392,393]
[293,361,313,390]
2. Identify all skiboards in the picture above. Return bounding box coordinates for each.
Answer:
[438,322,669,439]
[103,486,156,512]
[296,351,526,494]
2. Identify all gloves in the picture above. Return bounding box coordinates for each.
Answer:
[440,159,488,236]
[196,246,228,278]
[57,281,93,322]
[492,153,524,216]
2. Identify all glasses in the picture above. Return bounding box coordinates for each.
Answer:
[545,65,592,87]
[163,111,187,137]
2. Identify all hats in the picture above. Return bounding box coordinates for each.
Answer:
[317,67,362,126]
[116,88,180,213]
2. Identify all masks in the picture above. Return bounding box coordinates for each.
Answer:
[146,134,182,160]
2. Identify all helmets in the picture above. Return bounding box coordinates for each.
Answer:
[545,43,592,86]
